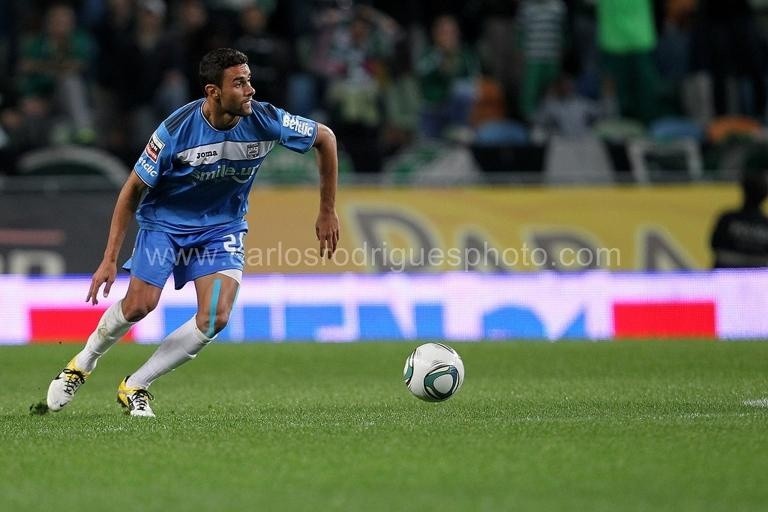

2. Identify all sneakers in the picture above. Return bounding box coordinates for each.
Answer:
[117,375,155,417]
[47,351,97,412]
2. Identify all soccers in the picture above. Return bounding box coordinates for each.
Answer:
[403,343,465,402]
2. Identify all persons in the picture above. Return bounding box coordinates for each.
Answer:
[711,155,768,269]
[1,1,767,184]
[46,48,341,418]
